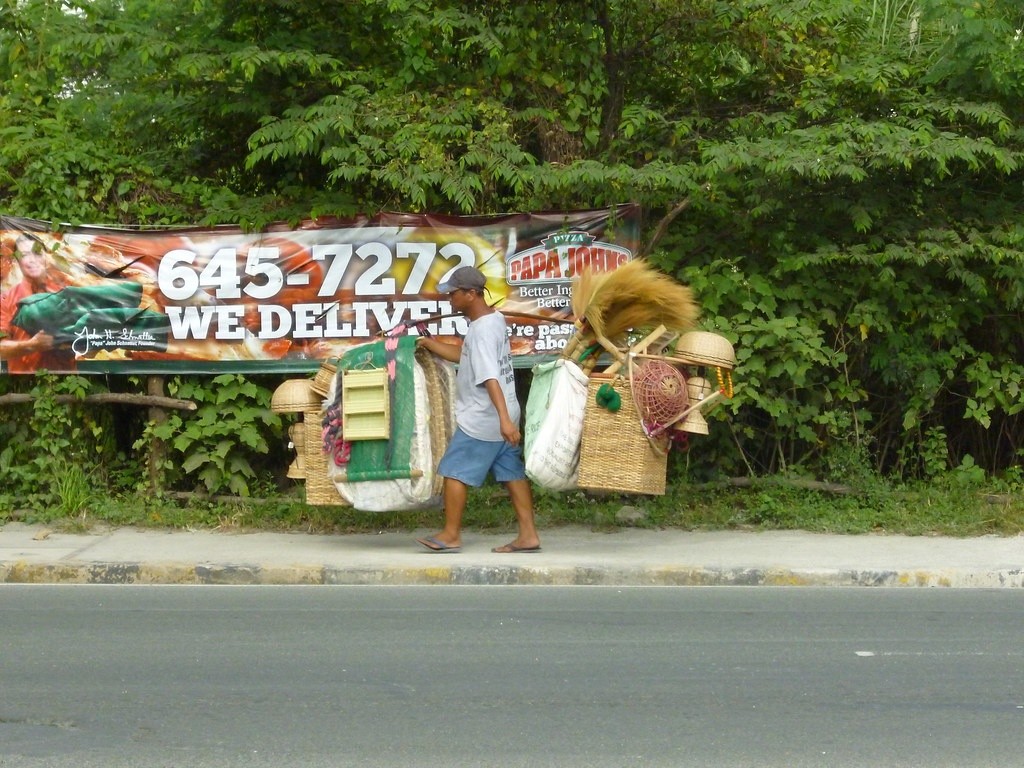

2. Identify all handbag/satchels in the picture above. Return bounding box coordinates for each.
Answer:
[524,359,589,491]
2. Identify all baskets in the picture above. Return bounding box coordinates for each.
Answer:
[272,356,353,505]
[633,332,734,435]
[578,377,669,495]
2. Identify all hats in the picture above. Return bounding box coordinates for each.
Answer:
[435,266,486,294]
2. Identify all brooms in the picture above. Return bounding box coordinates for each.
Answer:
[556,257,701,381]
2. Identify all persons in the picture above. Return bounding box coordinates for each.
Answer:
[0,233,78,372]
[414,265,542,554]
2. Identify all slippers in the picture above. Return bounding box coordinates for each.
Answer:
[491,543,542,553]
[414,536,461,553]
